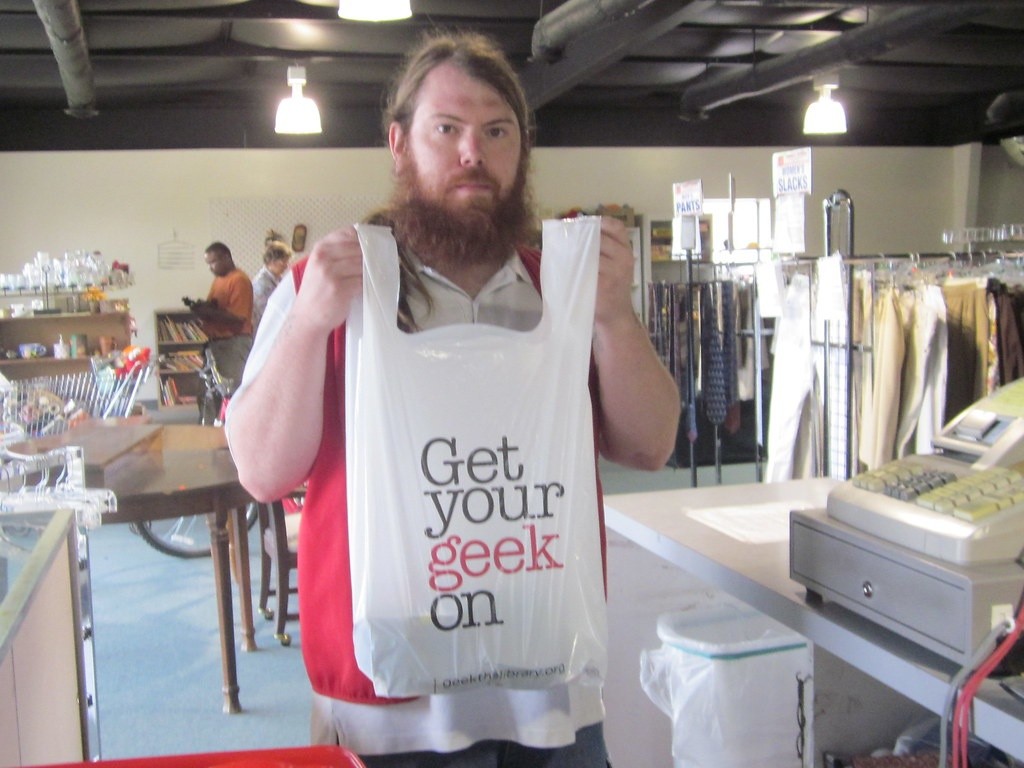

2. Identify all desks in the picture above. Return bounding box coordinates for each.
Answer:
[602,477,1024,768]
[7,421,255,714]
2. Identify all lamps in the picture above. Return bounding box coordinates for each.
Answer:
[803,74,847,134]
[274,66,323,135]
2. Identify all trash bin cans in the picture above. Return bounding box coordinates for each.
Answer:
[657,607,809,768]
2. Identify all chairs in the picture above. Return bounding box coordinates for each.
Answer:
[259,499,297,646]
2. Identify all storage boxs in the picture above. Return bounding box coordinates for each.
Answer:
[37,423,164,488]
[639,603,814,768]
[3,746,366,768]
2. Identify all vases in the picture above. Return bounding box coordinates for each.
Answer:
[89,300,100,313]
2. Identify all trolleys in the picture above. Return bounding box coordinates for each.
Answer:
[0,348,153,537]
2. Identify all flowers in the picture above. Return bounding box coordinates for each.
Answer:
[83,287,108,302]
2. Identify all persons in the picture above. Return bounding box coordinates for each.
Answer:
[222,35,683,768]
[203,230,292,399]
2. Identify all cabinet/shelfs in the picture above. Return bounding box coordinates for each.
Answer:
[0,287,130,382]
[0,498,103,768]
[156,312,209,408]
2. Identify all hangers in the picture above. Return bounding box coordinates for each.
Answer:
[647,245,1024,295]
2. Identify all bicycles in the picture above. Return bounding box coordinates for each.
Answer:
[125,348,257,560]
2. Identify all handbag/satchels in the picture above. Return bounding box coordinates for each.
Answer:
[347,216,612,698]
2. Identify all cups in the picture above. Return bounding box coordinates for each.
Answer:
[11,304,24,318]
[19,342,46,359]
[98,335,118,356]
[31,299,43,311]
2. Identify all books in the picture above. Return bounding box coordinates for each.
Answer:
[158,315,208,405]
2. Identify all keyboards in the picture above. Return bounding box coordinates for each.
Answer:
[852,457,1024,522]
